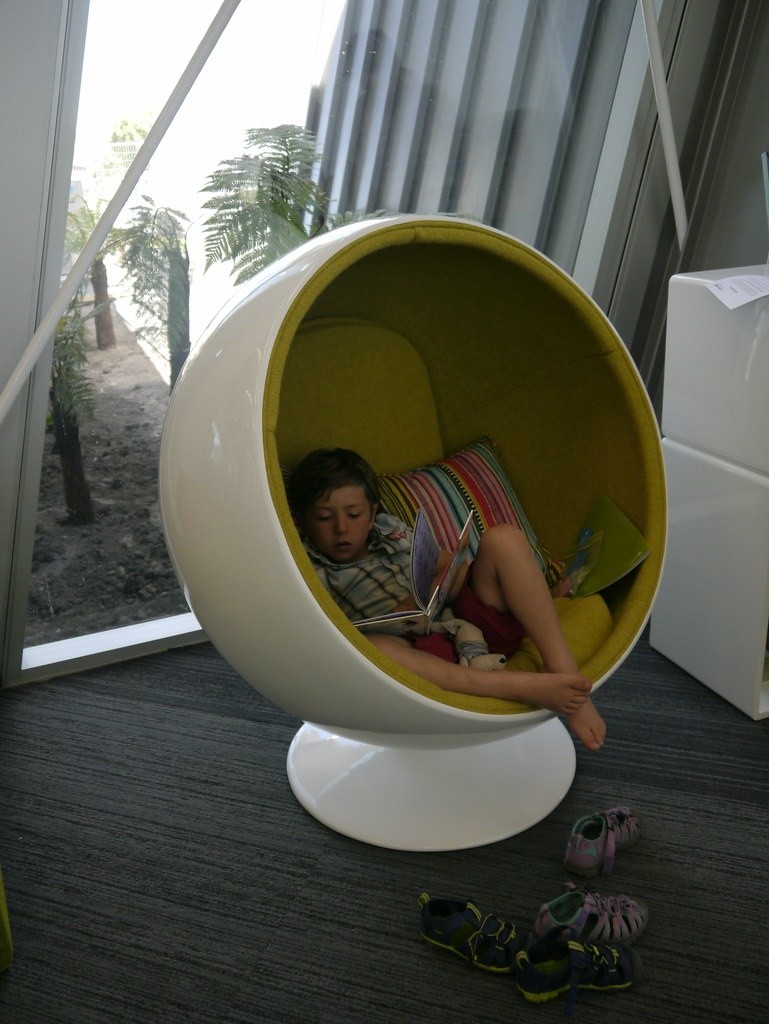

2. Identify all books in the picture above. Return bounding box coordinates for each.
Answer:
[352,508,475,637]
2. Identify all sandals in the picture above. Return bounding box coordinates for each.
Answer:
[513,925,640,1004]
[535,888,648,947]
[417,893,531,972]
[562,805,642,879]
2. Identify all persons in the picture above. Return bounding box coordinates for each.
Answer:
[282,448,608,753]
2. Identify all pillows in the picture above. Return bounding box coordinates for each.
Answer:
[377,434,566,590]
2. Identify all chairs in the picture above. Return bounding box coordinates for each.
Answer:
[157,212,672,854]
